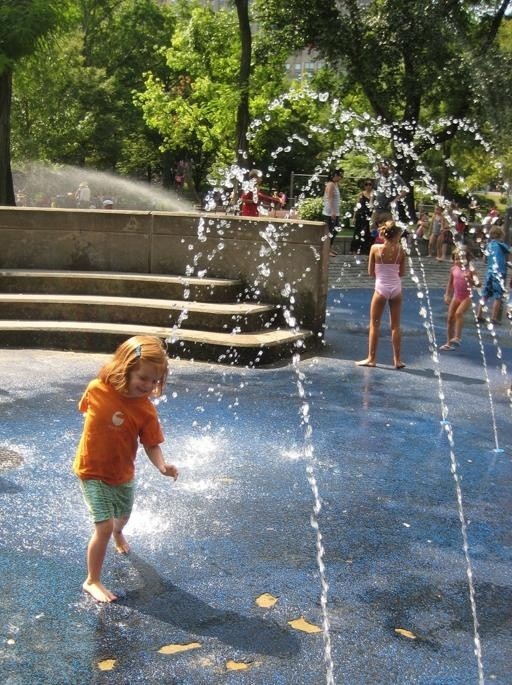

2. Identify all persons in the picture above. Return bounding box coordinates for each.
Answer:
[72,334,179,604]
[240,170,281,217]
[321,170,344,256]
[350,162,512,326]
[73,180,115,209]
[440,245,480,352]
[354,219,408,370]
